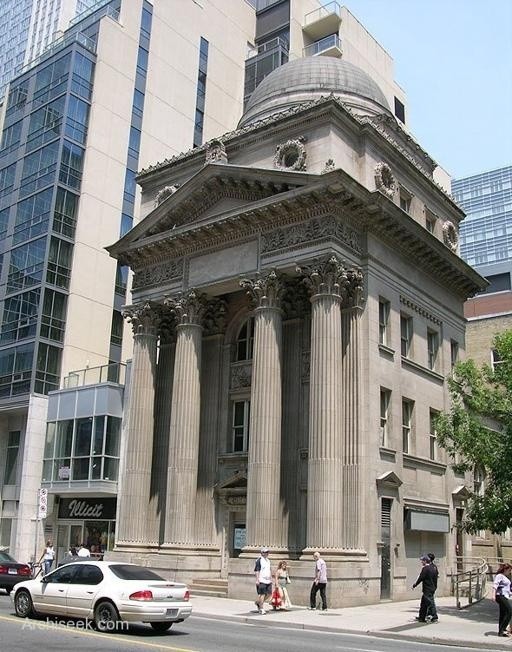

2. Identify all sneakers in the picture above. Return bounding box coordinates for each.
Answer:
[256,602,327,614]
[416,615,438,621]
[497,628,510,637]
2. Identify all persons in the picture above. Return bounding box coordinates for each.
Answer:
[68,529,103,558]
[275,561,291,610]
[254,549,272,615]
[37,540,55,575]
[413,555,438,622]
[308,552,328,610]
[493,563,511,637]
[423,553,438,619]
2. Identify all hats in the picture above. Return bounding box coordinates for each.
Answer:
[261,548,269,553]
[420,556,430,561]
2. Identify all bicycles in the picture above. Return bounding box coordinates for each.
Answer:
[28,561,47,580]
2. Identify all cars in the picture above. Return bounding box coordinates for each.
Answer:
[9,560,192,633]
[0,551,31,593]
